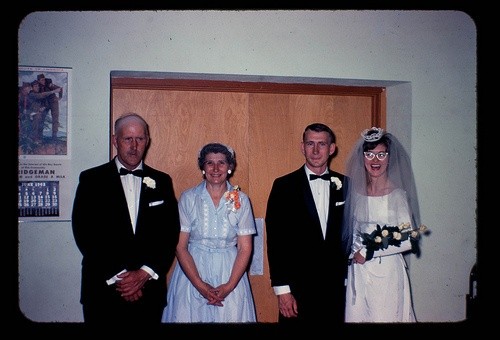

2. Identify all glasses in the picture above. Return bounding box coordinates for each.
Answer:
[363,151,388,160]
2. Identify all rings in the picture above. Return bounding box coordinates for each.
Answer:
[210,298,213,300]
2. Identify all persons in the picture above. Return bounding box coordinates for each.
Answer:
[19,74,64,140]
[72,114,180,324]
[265,123,352,322]
[161,142,258,323]
[341,126,420,323]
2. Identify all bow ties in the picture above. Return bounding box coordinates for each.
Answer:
[310,174,331,180]
[119,168,144,178]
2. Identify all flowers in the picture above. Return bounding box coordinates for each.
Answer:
[225,190,240,209]
[348,222,431,266]
